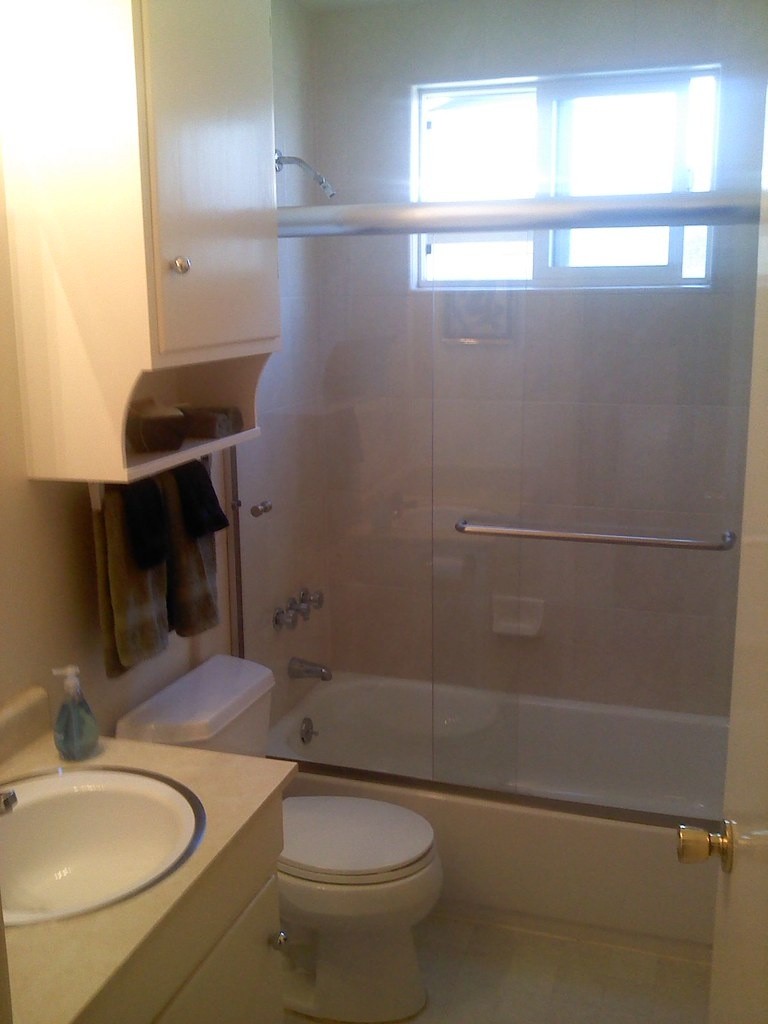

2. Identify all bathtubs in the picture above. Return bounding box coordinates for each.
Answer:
[263,669,731,948]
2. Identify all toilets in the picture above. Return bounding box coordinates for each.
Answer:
[113,651,447,1024]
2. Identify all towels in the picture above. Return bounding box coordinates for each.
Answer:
[148,403,243,439]
[90,459,234,671]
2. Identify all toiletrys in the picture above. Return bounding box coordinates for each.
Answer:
[51,663,103,762]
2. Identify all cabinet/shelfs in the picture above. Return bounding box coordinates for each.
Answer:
[86,796,288,1024]
[0,0,280,484]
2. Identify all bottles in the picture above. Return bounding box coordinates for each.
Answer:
[51,666,99,762]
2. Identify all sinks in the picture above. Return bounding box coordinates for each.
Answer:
[2,769,198,927]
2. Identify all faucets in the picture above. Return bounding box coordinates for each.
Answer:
[1,791,18,815]
[289,656,333,682]
